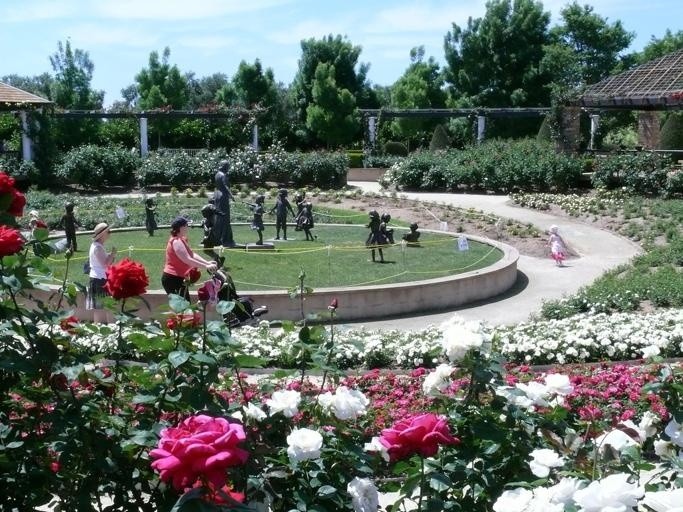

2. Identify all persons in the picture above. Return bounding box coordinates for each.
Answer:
[267,189,296,241]
[160,216,217,307]
[546,225,567,268]
[212,160,237,248]
[56,200,84,252]
[142,197,160,236]
[198,193,217,244]
[293,190,315,242]
[401,222,421,243]
[244,194,266,246]
[364,209,385,263]
[378,212,396,245]
[88,222,118,310]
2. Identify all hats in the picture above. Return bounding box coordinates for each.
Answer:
[172,217,188,229]
[549,226,558,234]
[92,222,115,242]
[215,256,225,269]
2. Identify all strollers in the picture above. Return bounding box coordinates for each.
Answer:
[206,263,269,329]
